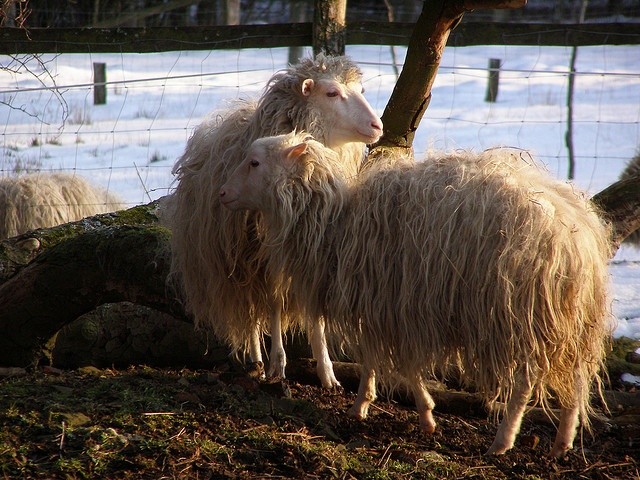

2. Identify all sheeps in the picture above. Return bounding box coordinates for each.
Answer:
[217,126,623,461]
[166,54,385,393]
[0,172,129,240]
